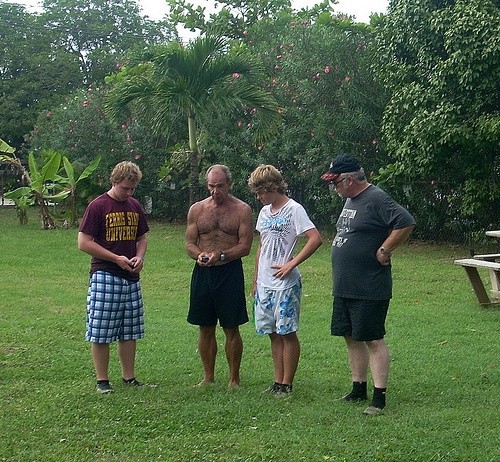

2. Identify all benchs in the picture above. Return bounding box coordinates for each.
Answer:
[455,259,500,307]
[474,254,500,261]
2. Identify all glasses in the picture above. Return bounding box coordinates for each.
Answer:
[329,178,346,191]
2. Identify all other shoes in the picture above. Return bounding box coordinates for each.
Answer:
[275,386,292,398]
[96,383,114,394]
[123,380,145,388]
[337,396,367,402]
[364,406,384,415]
[259,384,279,394]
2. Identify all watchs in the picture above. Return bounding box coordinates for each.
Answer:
[219,251,225,262]
[380,245,392,257]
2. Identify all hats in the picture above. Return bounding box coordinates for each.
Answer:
[320,155,361,181]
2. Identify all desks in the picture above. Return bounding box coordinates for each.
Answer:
[485,230,500,237]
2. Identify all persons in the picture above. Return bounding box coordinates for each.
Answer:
[186,164,254,395]
[77,162,156,394]
[247,164,323,398]
[320,156,415,416]
